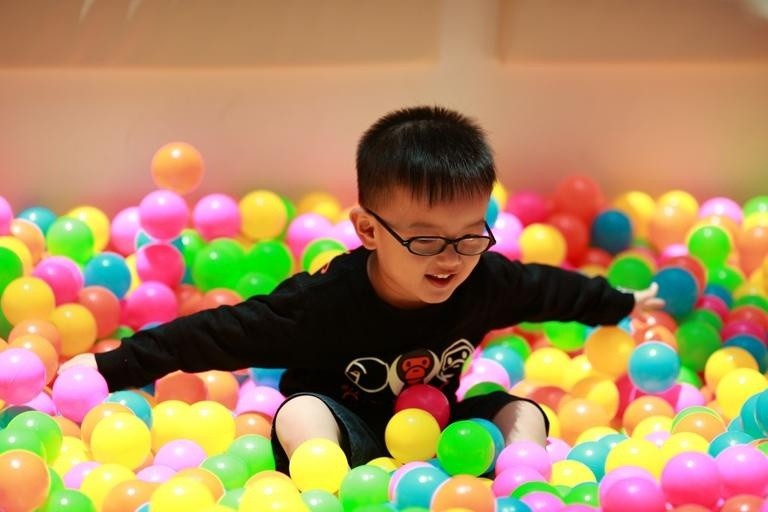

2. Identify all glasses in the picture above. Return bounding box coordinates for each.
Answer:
[366,209,497,257]
